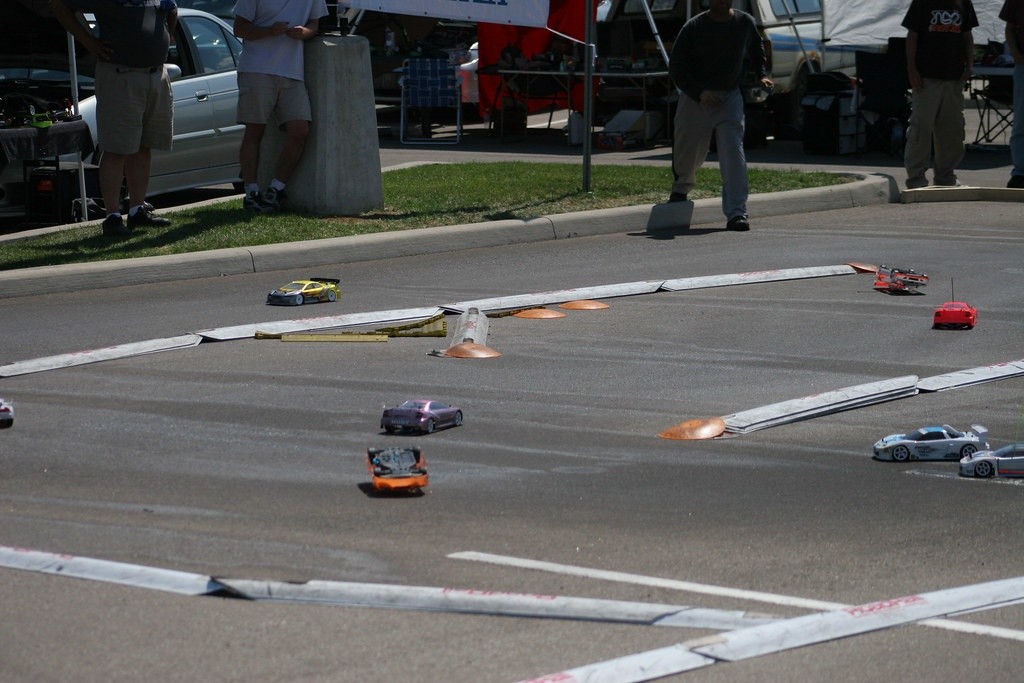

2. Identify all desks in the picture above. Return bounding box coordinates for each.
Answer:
[967,67,1015,144]
[0,118,94,225]
[493,69,673,149]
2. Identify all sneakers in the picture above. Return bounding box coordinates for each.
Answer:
[243,191,274,213]
[262,186,283,204]
[127,206,171,227]
[102,215,132,236]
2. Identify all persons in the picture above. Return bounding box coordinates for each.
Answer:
[901,0,979,190]
[667,0,773,231]
[998,0,1024,188]
[233,0,329,212]
[59,0,177,239]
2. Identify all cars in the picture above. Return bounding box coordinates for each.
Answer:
[0,8,244,201]
[594,0,823,142]
[960,444,1024,478]
[872,264,929,296]
[367,446,429,495]
[932,300,977,328]
[267,277,342,305]
[381,398,464,434]
[873,425,990,463]
[0,399,14,426]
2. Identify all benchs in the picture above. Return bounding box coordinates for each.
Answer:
[167,46,227,78]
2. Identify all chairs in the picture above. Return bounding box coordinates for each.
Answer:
[399,58,464,146]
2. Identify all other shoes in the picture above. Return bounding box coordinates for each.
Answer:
[1007,175,1024,188]
[952,178,967,186]
[727,216,749,230]
